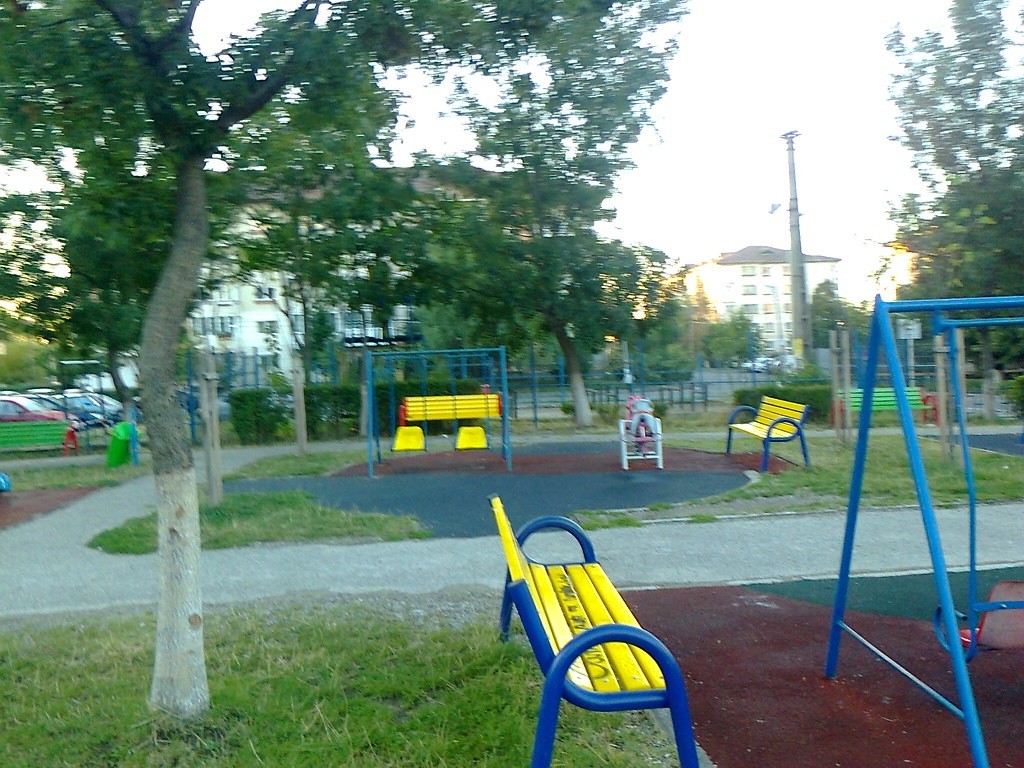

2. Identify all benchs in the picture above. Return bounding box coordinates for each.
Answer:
[486,493,699,768]
[0,420,74,452]
[831,387,938,427]
[726,395,812,473]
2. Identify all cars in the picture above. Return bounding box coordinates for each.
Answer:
[730,355,794,373]
[1,388,141,432]
[0,396,84,432]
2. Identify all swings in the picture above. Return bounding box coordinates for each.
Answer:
[387,355,427,452]
[934,315,1024,663]
[449,352,491,450]
